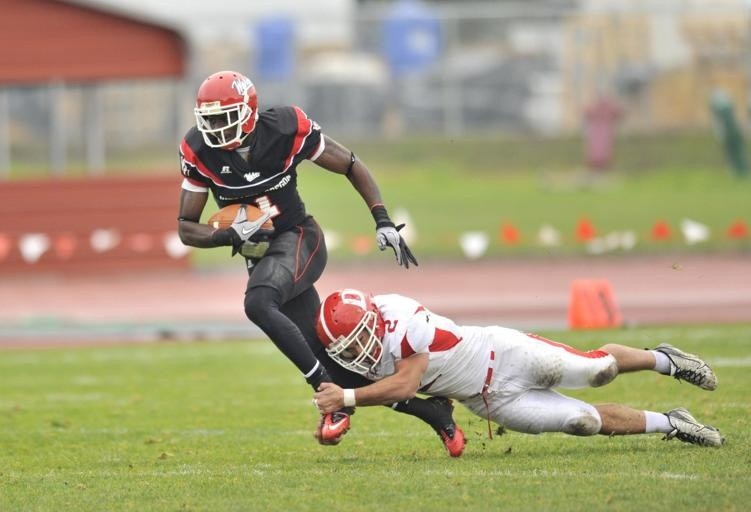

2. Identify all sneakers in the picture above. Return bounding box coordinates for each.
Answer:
[314,410,351,446]
[654,342,717,391]
[426,396,467,457]
[663,407,726,449]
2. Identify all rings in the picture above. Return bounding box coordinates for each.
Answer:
[312,399,318,405]
[315,405,319,409]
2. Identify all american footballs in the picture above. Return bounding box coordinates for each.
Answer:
[208,204,273,229]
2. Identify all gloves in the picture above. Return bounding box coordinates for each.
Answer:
[375,221,419,270]
[211,203,276,257]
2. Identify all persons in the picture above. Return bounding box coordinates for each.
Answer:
[175,69,469,459]
[310,286,729,450]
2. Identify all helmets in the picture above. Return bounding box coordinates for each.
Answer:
[313,289,385,376]
[193,71,259,152]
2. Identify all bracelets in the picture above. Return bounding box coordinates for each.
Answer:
[343,389,356,407]
